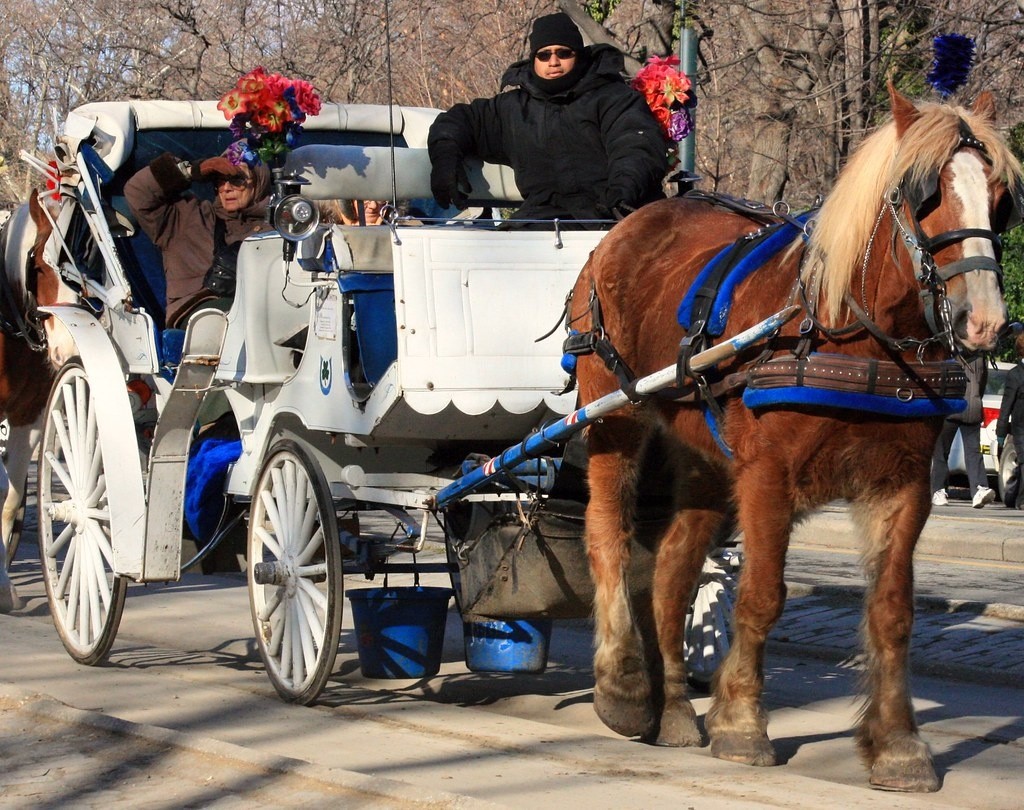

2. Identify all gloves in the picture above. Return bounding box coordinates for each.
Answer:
[594,178,639,225]
[191,156,239,182]
[428,143,473,211]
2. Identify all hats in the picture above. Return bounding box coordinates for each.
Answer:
[529,12,584,53]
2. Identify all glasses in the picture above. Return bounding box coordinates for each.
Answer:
[534,49,571,62]
[217,176,248,193]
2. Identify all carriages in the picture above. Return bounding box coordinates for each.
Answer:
[35,71,1024,797]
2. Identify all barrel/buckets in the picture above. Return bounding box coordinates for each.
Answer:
[464,615,552,674]
[345,522,455,681]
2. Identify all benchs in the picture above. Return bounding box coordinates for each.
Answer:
[104,231,186,377]
[283,145,525,270]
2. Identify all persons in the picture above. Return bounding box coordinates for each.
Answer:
[995,332,1024,511]
[314,194,462,226]
[123,143,275,331]
[928,350,996,508]
[428,13,666,231]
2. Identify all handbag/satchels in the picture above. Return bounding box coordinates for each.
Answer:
[203,240,242,298]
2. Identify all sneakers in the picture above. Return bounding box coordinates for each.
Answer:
[972,485,996,509]
[932,488,949,506]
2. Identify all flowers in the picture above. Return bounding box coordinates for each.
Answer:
[631,54,697,141]
[216,65,322,169]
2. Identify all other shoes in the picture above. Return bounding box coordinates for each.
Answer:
[1005,496,1016,509]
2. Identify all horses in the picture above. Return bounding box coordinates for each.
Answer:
[566,70,1024,793]
[0,187,87,614]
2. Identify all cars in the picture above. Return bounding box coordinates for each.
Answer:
[990,433,1018,506]
[948,362,1018,487]
